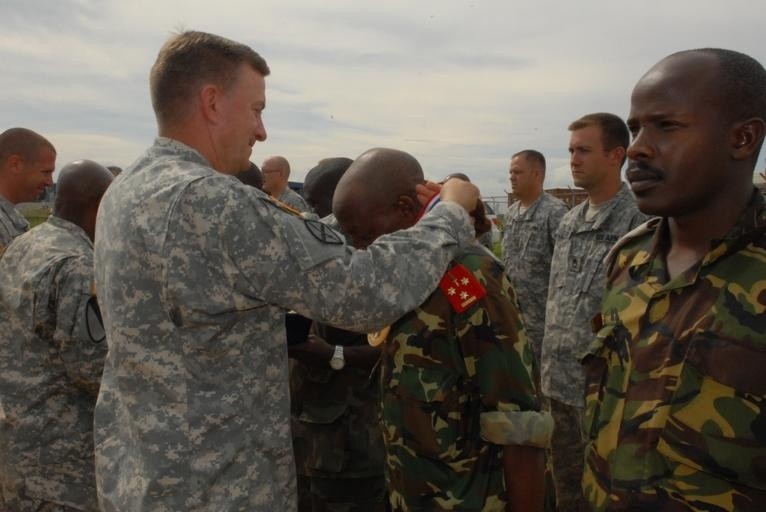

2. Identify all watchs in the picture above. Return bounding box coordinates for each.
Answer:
[329,344,346,373]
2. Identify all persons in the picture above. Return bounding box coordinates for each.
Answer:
[575,48,766,512]
[289,157,382,512]
[539,112,649,512]
[501,150,570,410]
[261,156,309,213]
[93,32,480,512]
[0,126,57,258]
[440,173,493,252]
[0,159,115,512]
[332,147,554,512]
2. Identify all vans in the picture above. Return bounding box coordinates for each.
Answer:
[482,199,500,243]
